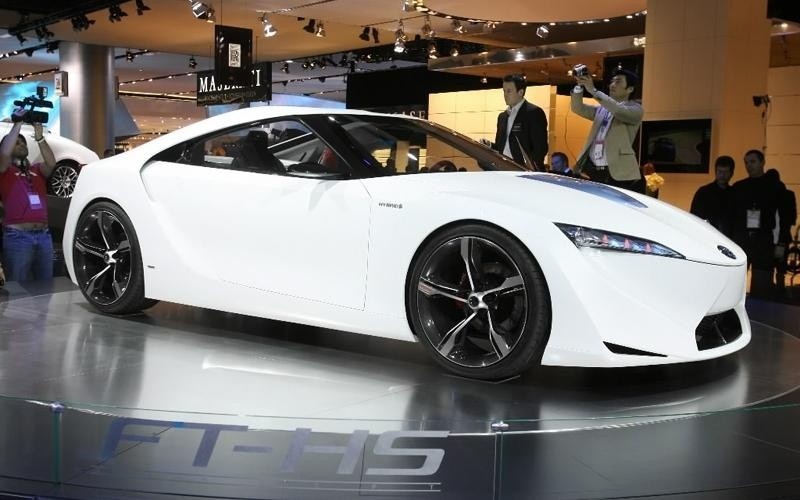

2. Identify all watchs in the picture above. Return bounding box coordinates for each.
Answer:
[592,88,598,95]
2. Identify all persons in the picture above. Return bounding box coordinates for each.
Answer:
[733,149,786,300]
[690,156,735,240]
[0,108,57,281]
[570,68,645,194]
[765,168,797,289]
[480,73,548,173]
[551,152,573,177]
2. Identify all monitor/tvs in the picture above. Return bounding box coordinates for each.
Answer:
[638,118,713,175]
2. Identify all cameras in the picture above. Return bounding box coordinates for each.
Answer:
[572,63,588,77]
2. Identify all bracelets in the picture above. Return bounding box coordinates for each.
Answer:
[37,136,45,143]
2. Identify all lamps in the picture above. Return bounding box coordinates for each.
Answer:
[449,44,460,58]
[402,2,497,38]
[536,25,550,38]
[191,2,216,23]
[258,11,278,38]
[428,41,439,60]
[1,1,152,59]
[394,29,408,53]
[477,45,488,57]
[359,26,379,43]
[302,19,326,38]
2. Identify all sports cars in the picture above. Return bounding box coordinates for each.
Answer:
[20,301,453,500]
[64,104,753,382]
[0,121,100,202]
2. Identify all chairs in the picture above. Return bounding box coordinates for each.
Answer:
[228,127,288,175]
